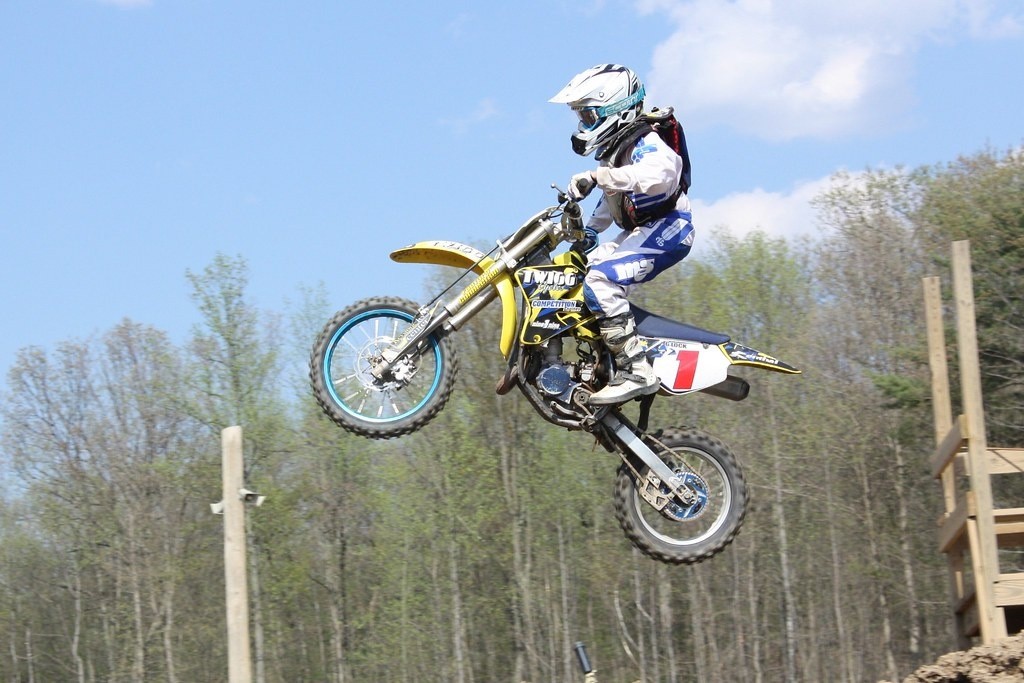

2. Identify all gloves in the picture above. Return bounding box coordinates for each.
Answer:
[567,170,597,202]
[570,229,599,254]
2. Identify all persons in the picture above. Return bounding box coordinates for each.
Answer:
[547,64,695,403]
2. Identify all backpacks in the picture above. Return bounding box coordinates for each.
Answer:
[613,106,691,232]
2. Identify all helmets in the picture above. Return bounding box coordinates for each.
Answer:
[547,63,645,161]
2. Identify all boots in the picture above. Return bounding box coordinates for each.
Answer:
[589,310,659,404]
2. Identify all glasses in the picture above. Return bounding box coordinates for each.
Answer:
[578,107,601,131]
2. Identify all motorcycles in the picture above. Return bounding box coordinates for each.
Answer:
[308,178,803,565]
